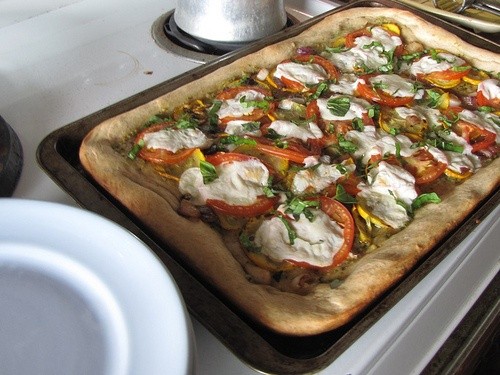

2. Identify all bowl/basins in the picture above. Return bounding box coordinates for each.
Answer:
[0,197,198,375]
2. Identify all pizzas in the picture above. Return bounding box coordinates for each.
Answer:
[78,7,500,336]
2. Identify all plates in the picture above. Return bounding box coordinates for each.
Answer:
[401,0,500,34]
